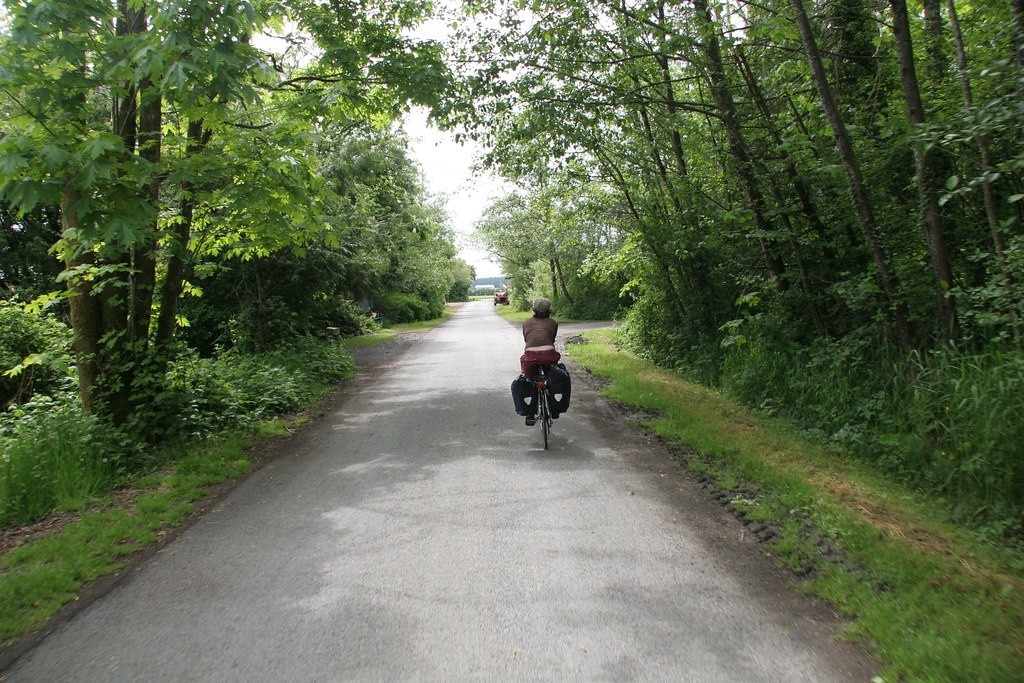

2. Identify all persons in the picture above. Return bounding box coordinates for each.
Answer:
[520,297,561,426]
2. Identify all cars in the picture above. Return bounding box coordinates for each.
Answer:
[493,291,510,307]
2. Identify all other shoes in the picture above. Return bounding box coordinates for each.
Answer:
[552,412,559,419]
[525,416,535,426]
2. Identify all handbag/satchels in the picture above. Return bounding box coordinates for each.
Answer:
[548,363,571,412]
[510,374,538,415]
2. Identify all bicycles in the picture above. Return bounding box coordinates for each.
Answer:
[528,364,555,450]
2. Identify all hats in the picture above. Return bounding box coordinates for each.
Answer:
[532,297,550,313]
